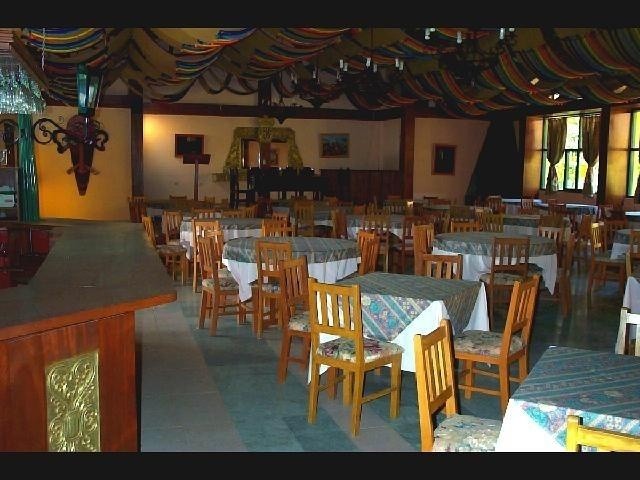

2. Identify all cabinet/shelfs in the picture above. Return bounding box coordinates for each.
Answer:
[0,166,24,224]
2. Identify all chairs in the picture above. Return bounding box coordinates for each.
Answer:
[228,166,329,209]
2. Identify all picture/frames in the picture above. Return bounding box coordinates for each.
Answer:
[175,133,204,156]
[434,145,457,174]
[318,133,350,158]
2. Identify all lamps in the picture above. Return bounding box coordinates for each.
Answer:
[300,58,342,108]
[423,27,507,91]
[259,73,302,124]
[75,62,104,117]
[344,28,401,104]
